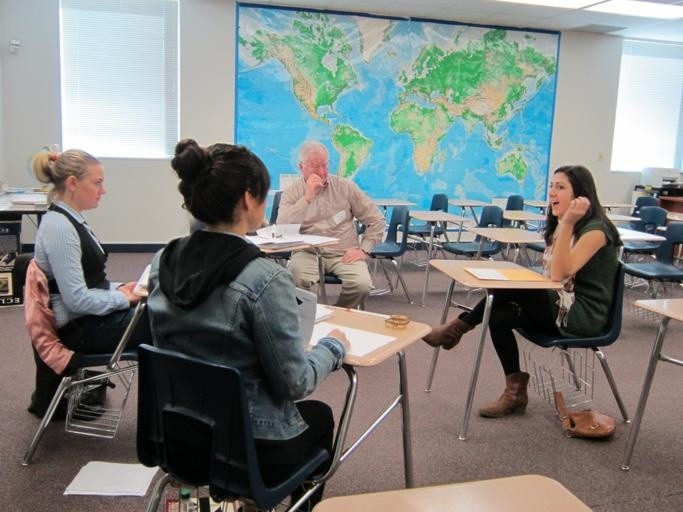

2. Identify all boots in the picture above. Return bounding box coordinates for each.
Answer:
[478,372,530,417]
[422,311,475,350]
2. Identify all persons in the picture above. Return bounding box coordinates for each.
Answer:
[147,137,350,512]
[422,163,620,418]
[276,140,386,306]
[29,145,147,349]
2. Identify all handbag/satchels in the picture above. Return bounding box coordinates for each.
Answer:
[29,345,115,420]
[556,391,616,437]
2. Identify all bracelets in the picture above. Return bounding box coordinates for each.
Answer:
[362,249,369,256]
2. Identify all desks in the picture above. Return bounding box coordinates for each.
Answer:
[0,187,47,304]
